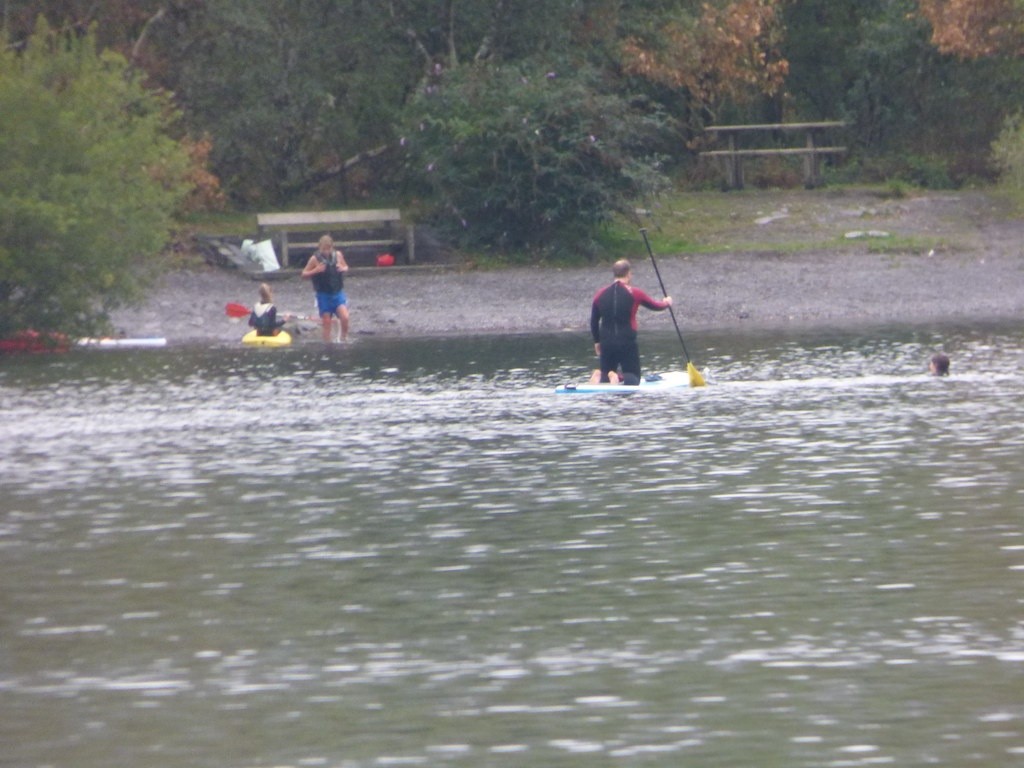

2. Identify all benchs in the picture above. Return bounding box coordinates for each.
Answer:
[277,223,416,268]
[698,146,849,190]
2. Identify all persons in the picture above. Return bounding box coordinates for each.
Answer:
[590,260,673,385]
[302,236,352,343]
[249,284,291,336]
[928,354,949,377]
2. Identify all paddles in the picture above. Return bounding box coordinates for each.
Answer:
[225,300,315,324]
[635,223,708,387]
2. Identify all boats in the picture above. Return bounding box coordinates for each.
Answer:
[242,326,291,348]
[0,327,167,355]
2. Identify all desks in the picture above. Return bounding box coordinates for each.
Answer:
[254,209,402,241]
[704,116,847,190]
